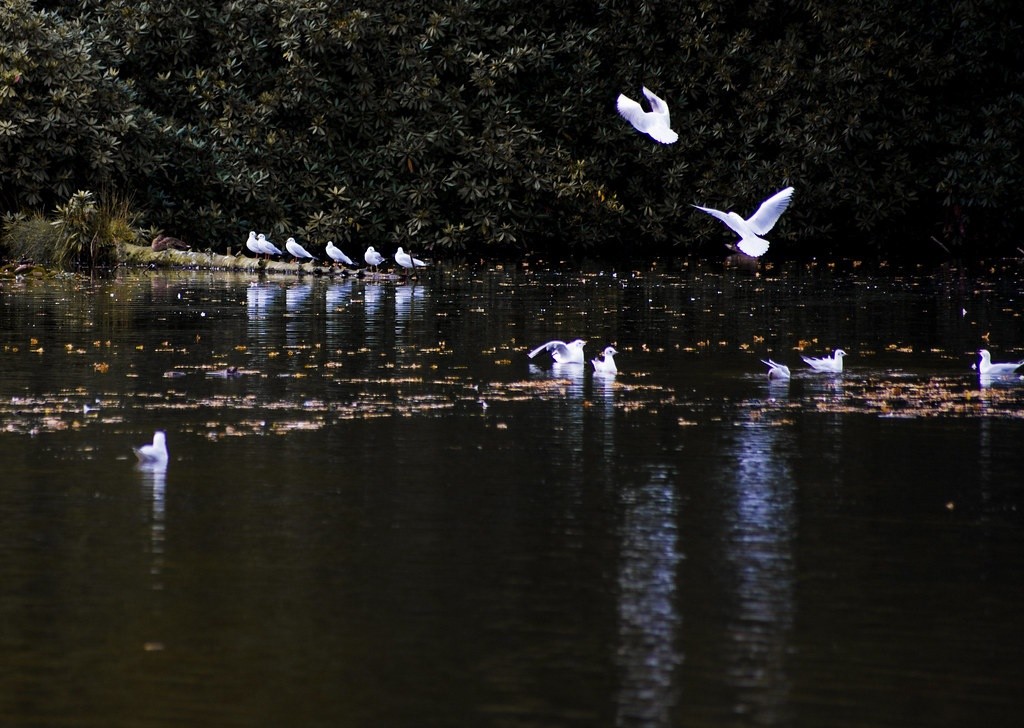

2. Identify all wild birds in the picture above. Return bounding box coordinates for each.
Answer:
[617,85,679,144]
[800,349,849,371]
[690,186,795,258]
[977,350,1024,374]
[246,230,427,270]
[132,430,168,460]
[760,359,791,378]
[528,339,620,377]
